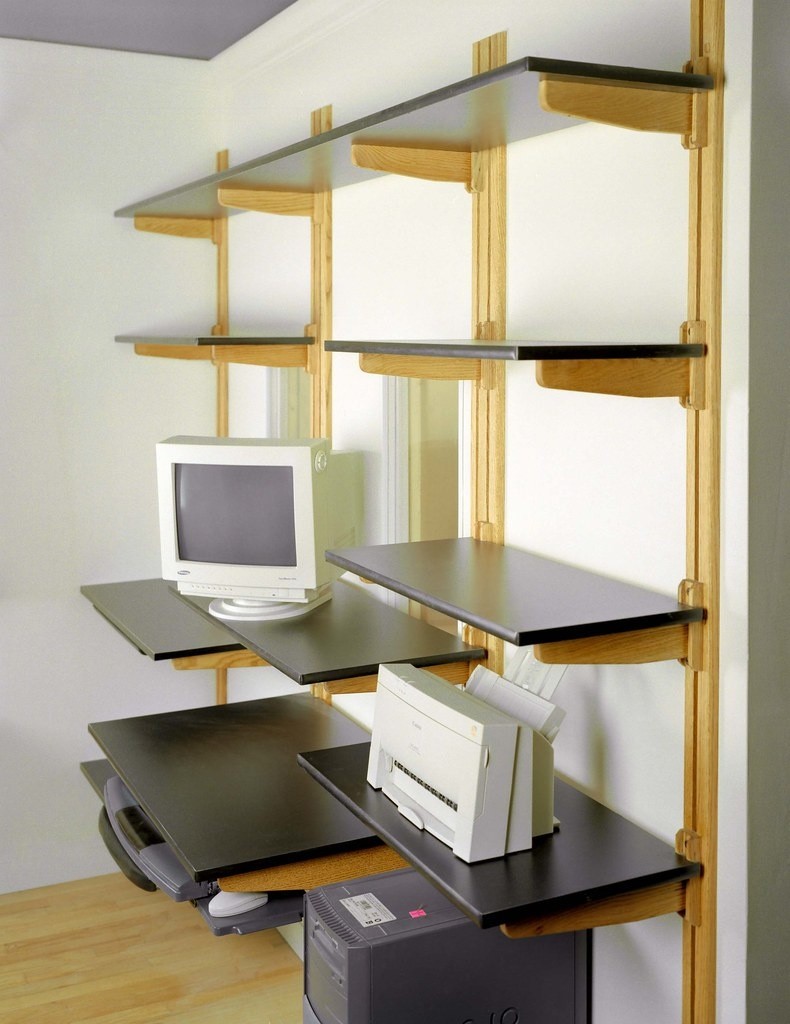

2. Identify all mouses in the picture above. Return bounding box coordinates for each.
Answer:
[208,890,268,918]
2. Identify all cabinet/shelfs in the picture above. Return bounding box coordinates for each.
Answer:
[80,0,725,1024]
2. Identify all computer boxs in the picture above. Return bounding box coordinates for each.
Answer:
[301,867,590,1024]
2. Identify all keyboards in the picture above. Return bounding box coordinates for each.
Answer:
[104,776,219,902]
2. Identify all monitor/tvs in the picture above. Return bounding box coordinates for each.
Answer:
[157,437,364,622]
